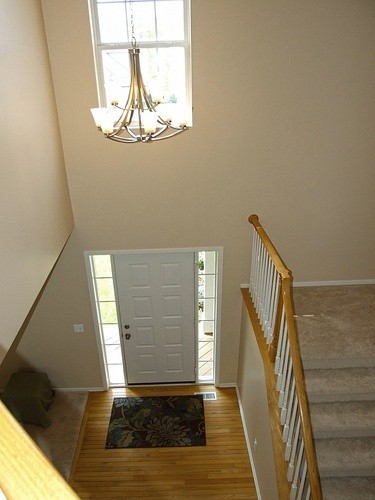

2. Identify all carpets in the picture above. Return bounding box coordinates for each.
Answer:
[103,394,206,448]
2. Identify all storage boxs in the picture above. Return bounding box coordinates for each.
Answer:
[4,371,57,426]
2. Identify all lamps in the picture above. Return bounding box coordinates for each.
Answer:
[89,0,194,144]
